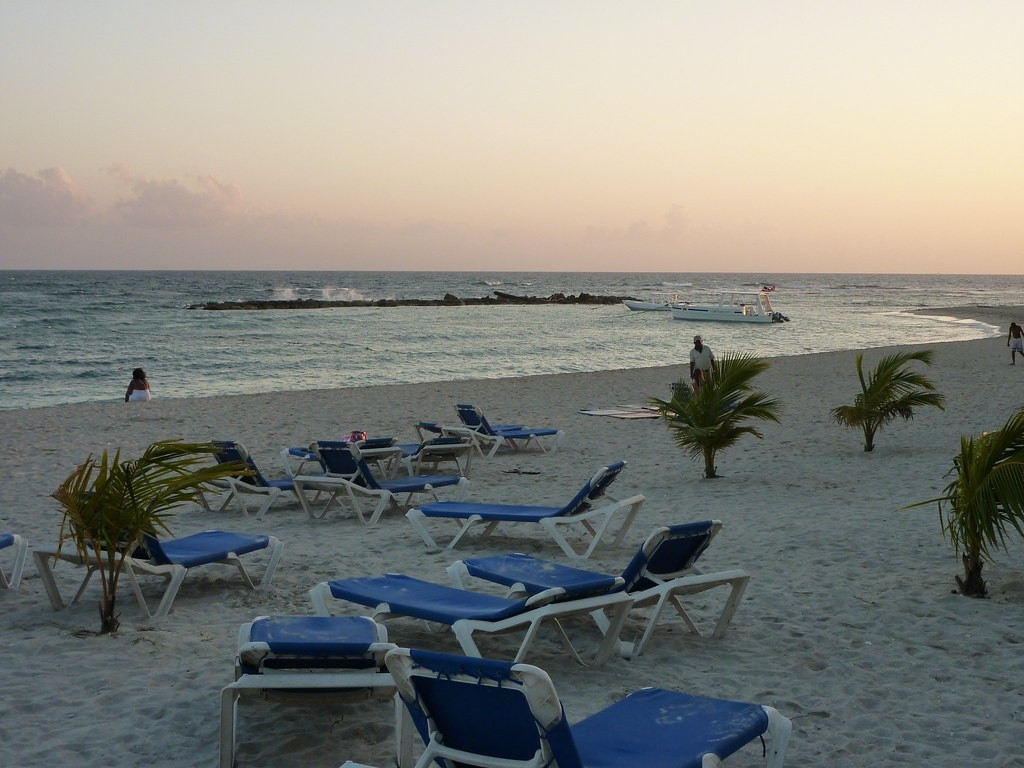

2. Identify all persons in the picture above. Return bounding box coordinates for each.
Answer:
[739,304,746,316]
[125,368,152,403]
[1007,322,1024,365]
[689,336,717,396]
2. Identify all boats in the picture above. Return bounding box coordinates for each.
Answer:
[621,284,790,323]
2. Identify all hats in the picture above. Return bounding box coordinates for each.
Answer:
[692,335,705,343]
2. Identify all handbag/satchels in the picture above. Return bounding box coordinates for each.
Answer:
[346,430,368,442]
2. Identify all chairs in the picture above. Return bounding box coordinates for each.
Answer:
[447,520,750,667]
[280,436,404,481]
[0,534,29,589]
[441,403,565,461]
[33,529,284,619]
[309,567,634,664]
[385,648,792,768]
[220,617,414,768]
[293,440,471,525]
[386,437,471,480]
[405,461,646,559]
[192,442,326,520]
[413,421,522,455]
